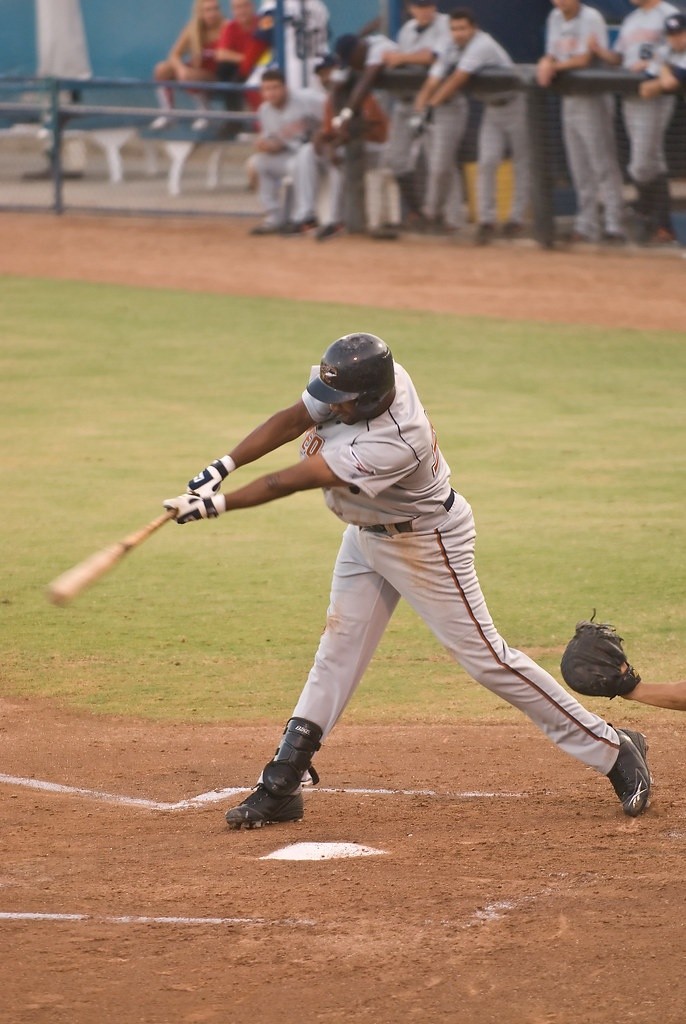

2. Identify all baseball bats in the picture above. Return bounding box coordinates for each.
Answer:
[46,484,221,607]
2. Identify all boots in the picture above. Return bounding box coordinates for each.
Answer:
[630,173,673,244]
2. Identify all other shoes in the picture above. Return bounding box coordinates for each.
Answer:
[317,221,345,241]
[249,220,285,236]
[191,118,209,131]
[563,230,591,243]
[474,224,499,245]
[400,212,434,232]
[502,223,520,239]
[281,217,319,238]
[372,224,397,240]
[423,216,456,233]
[602,229,625,246]
[151,116,171,129]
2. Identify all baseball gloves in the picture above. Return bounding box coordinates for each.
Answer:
[560,619,642,700]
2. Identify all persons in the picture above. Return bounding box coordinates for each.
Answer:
[617,661,686,712]
[164,333,653,828]
[33,0,686,252]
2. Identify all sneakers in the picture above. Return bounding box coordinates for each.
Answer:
[607,723,653,818]
[225,784,304,828]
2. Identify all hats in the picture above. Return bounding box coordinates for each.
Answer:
[661,15,686,33]
[312,51,341,73]
[335,33,357,70]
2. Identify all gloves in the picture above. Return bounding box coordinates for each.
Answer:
[162,492,226,524]
[187,454,236,501]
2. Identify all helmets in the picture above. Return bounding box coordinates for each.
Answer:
[307,333,396,419]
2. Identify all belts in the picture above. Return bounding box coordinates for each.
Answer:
[359,489,455,534]
